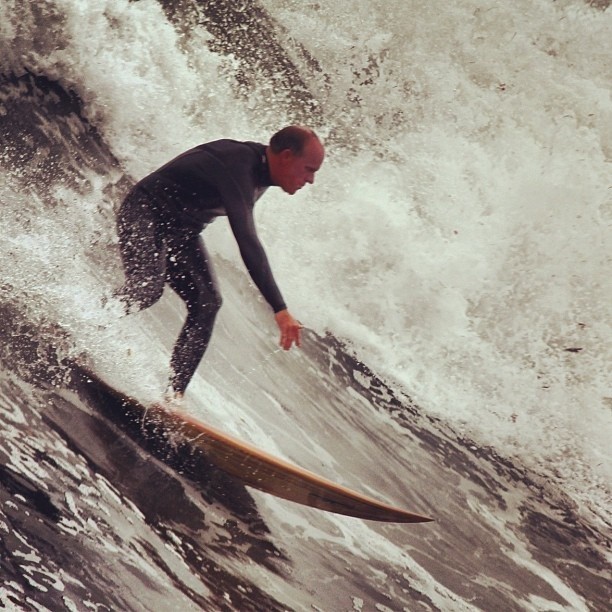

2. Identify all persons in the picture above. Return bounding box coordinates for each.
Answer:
[100,126,325,404]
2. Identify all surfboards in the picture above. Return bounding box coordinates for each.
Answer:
[79,365,434,522]
